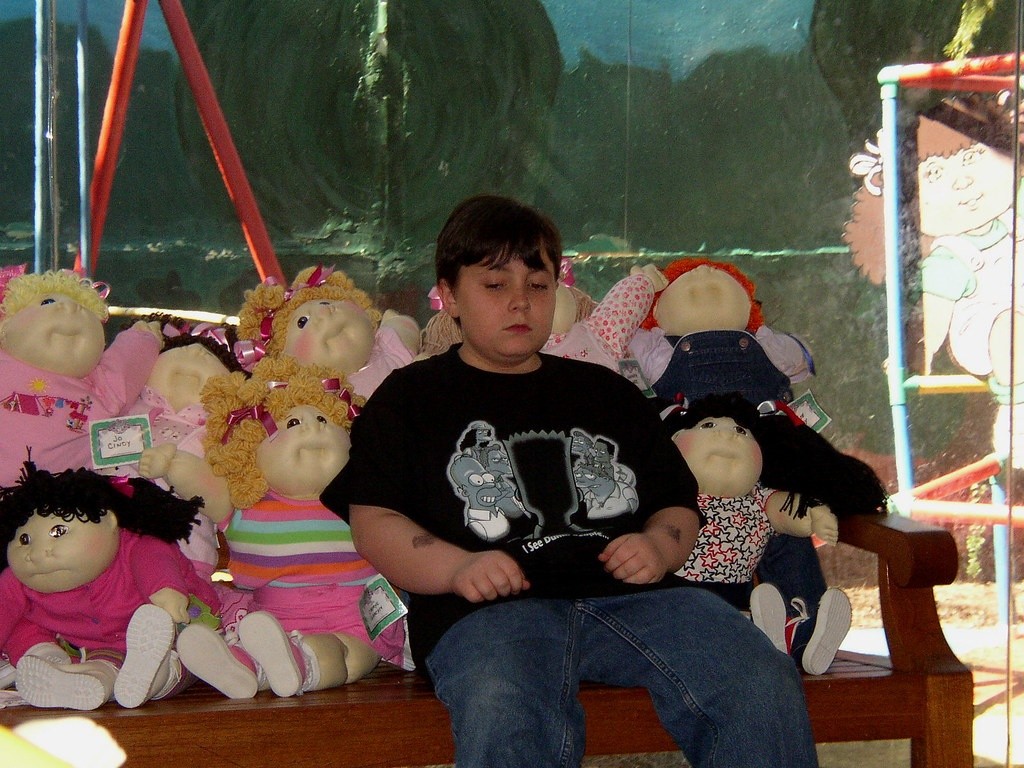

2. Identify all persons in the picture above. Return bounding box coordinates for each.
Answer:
[420,252,668,375]
[656,389,890,675]
[632,255,820,407]
[0,458,219,711]
[139,355,404,699]
[318,194,821,768]
[121,314,252,584]
[239,266,422,405]
[0,265,166,489]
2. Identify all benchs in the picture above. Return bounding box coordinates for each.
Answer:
[0,512,976,768]
